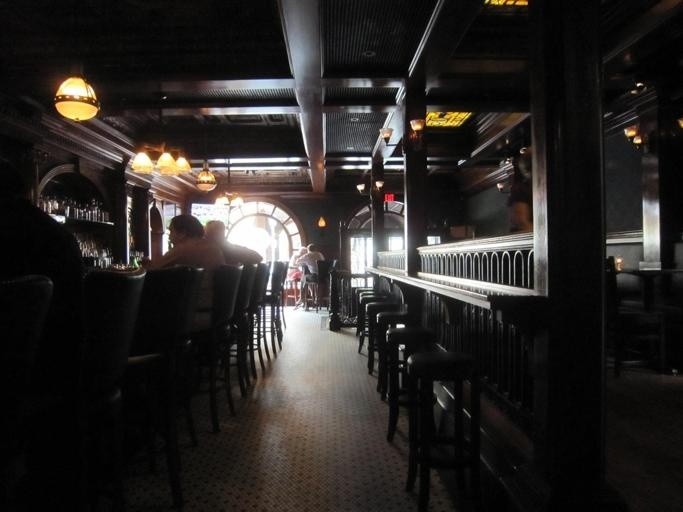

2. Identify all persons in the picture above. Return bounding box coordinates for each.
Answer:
[0,154,82,484]
[287,246,313,298]
[295,243,325,306]
[506,174,533,289]
[202,218,264,354]
[137,212,228,394]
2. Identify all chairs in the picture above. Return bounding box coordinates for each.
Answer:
[286,279,298,307]
[183,261,288,435]
[605,256,669,377]
[305,259,338,312]
[125,264,190,511]
[3,277,55,509]
[46,261,146,511]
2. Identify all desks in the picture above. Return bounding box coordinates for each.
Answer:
[609,269,682,312]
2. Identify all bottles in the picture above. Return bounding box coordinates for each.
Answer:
[37,193,112,224]
[76,232,144,268]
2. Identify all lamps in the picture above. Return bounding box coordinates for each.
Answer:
[379,128,404,148]
[128,90,218,193]
[356,184,370,196]
[375,181,384,191]
[53,71,101,121]
[624,125,651,156]
[409,119,425,140]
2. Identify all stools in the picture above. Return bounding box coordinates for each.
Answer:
[353,286,482,512]
